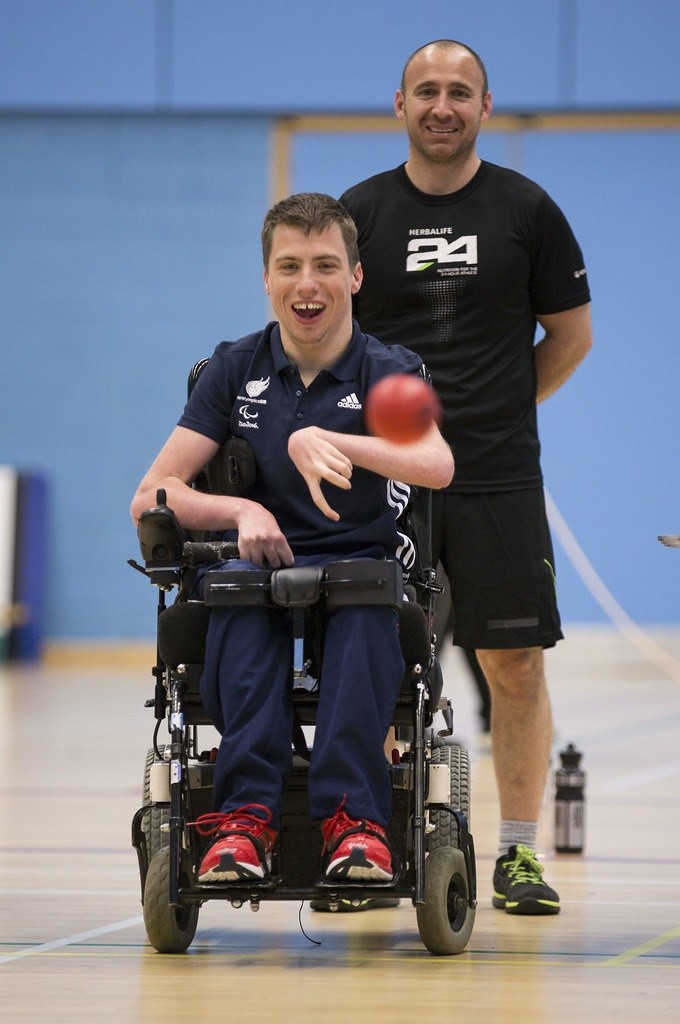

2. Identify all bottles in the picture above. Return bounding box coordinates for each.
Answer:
[554,743,585,853]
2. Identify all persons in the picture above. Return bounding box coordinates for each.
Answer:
[309,38,592,916]
[130,193,454,883]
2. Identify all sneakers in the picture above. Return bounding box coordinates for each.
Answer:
[197,814,278,884]
[491,845,561,916]
[309,897,400,912]
[322,817,395,883]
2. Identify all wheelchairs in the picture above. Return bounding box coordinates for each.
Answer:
[128,357,478,956]
